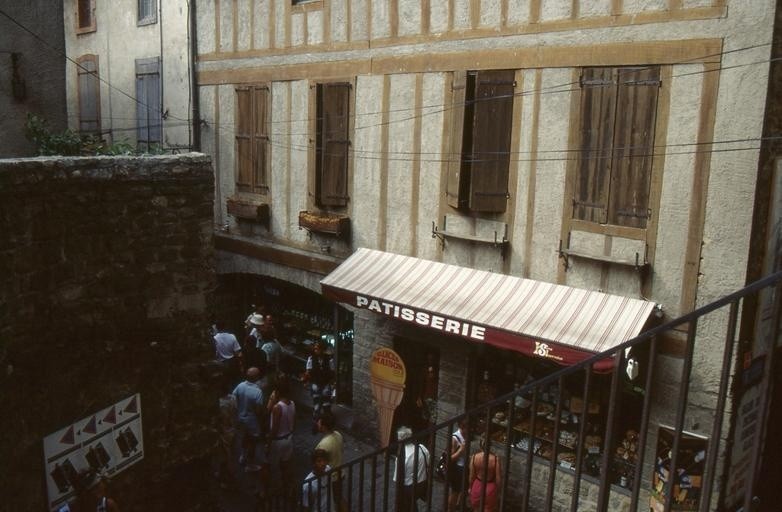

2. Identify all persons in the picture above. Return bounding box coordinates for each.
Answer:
[416,352,436,425]
[58,473,119,512]
[448,417,501,512]
[396,427,430,512]
[213,314,347,512]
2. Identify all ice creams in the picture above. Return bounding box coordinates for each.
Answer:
[370,348,406,458]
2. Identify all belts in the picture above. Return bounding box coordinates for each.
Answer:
[275,433,290,440]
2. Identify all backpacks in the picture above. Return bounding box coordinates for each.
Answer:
[434,435,461,483]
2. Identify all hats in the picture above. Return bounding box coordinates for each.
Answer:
[250,314,264,325]
